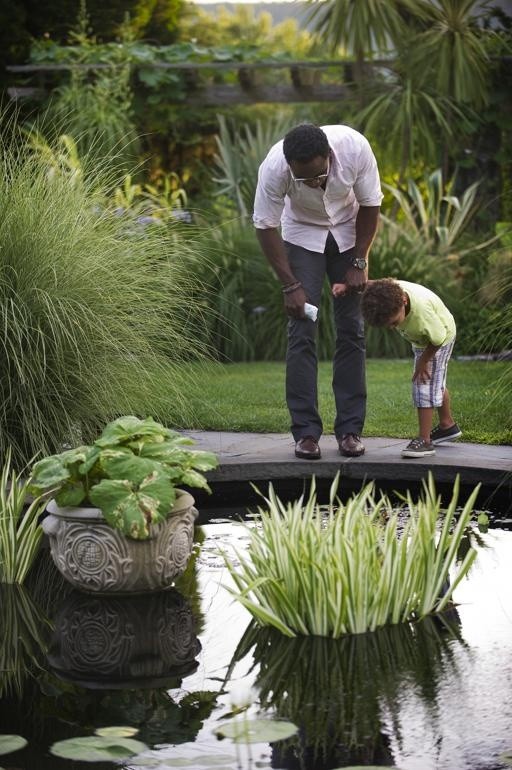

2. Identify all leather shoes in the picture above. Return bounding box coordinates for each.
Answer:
[295,438,320,459]
[338,433,364,456]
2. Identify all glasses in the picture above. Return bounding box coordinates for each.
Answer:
[287,161,329,185]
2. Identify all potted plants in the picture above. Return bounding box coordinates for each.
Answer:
[29,413,218,596]
[22,598,230,748]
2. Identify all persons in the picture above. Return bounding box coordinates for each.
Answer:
[251,122,385,460]
[331,276,464,458]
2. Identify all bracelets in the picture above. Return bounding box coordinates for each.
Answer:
[281,280,302,293]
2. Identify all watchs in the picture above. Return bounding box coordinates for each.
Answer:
[351,256,367,271]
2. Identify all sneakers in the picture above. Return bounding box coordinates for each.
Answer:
[430,425,462,445]
[401,437,436,458]
